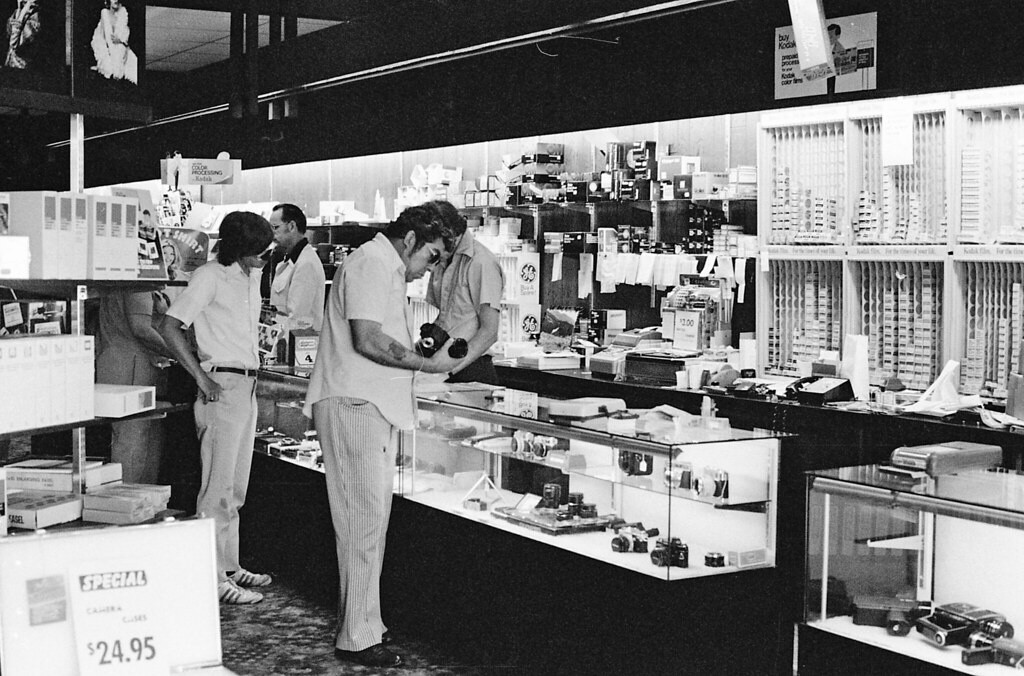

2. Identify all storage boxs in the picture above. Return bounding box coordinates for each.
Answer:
[89,195,140,281]
[0,333,95,433]
[10,190,88,281]
[50,236,56,239]
[2,458,172,529]
[95,382,156,417]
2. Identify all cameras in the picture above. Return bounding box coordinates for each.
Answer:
[509,430,559,461]
[611,526,648,554]
[415,322,468,361]
[650,537,688,568]
[557,491,598,522]
[853,595,1024,669]
[664,460,729,502]
[618,450,653,476]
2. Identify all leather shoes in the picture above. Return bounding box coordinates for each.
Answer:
[383,630,405,645]
[335,644,404,668]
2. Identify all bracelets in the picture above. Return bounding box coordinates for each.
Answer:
[447,372,453,378]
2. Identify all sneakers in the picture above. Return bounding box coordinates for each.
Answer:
[229,568,273,587]
[218,579,263,604]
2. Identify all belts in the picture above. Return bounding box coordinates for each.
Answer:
[477,355,492,363]
[210,365,260,377]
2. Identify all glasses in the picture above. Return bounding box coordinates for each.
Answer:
[252,248,272,261]
[271,223,287,230]
[420,240,441,267]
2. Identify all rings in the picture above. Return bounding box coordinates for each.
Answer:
[210,398,214,401]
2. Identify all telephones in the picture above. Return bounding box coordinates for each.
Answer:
[785,375,855,405]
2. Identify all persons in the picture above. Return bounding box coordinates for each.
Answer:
[827,24,845,52]
[303,206,465,669]
[160,239,182,280]
[259,335,268,349]
[421,200,506,386]
[97,203,325,605]
[90,0,130,80]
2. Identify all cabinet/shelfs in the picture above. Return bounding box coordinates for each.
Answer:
[0,277,188,533]
[252,355,800,676]
